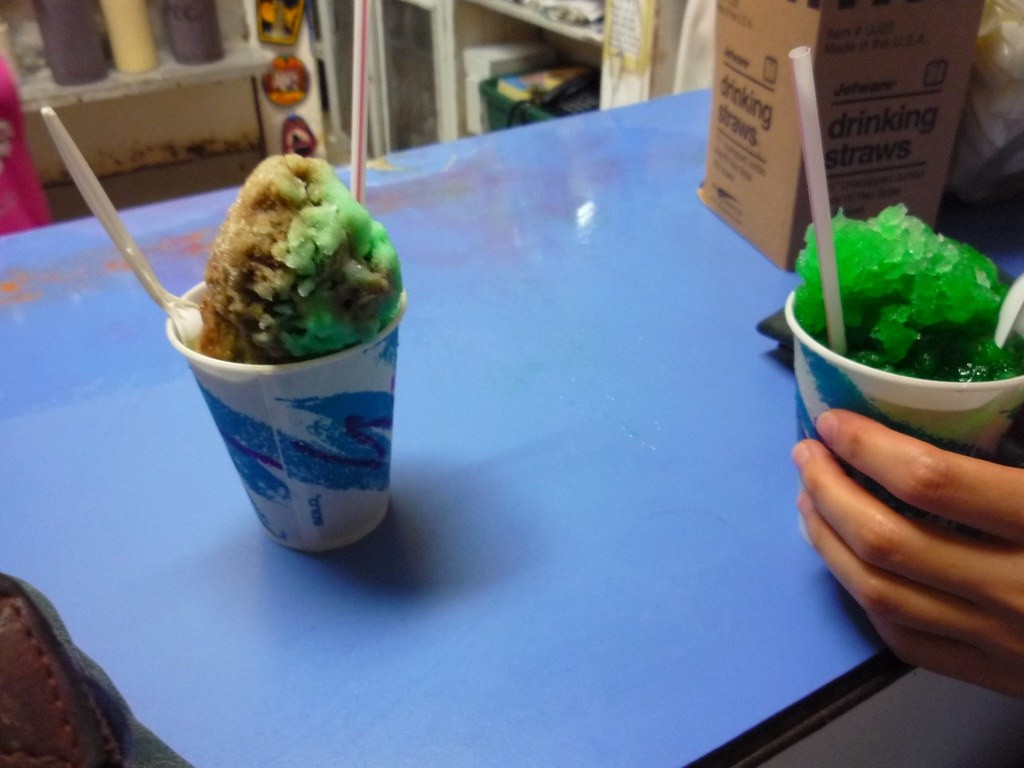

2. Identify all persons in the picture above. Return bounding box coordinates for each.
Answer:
[791,409,1024,692]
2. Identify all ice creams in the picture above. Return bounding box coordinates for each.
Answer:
[197,154,402,364]
[794,201,1024,382]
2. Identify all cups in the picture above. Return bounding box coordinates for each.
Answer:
[33,0,109,86]
[166,283,408,551]
[161,0,224,65]
[783,291,1024,621]
[99,0,158,74]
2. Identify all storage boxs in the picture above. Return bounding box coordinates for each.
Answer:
[700,0,986,273]
[479,64,601,137]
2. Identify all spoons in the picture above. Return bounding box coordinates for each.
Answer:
[40,105,204,355]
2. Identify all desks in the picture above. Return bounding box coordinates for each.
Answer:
[1,91,1023,766]
